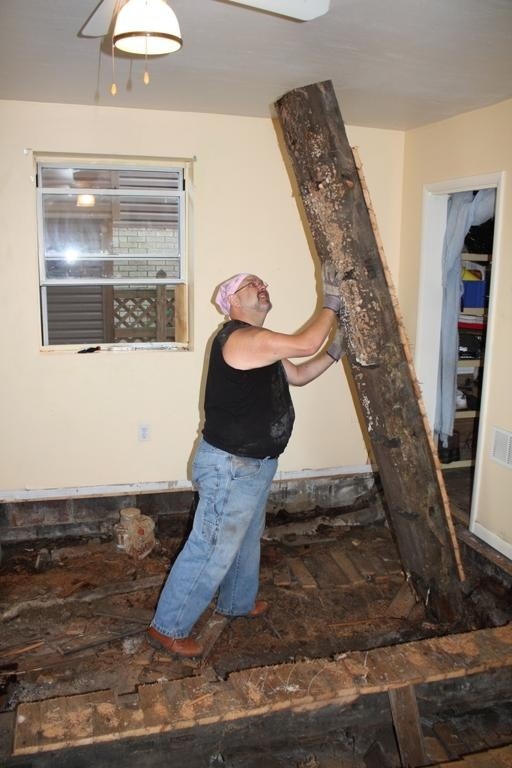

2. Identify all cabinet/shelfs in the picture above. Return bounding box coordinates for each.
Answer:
[439,253,493,470]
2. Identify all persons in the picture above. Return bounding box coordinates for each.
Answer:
[143,257,357,658]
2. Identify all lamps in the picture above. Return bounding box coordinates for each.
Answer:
[112,0,183,57]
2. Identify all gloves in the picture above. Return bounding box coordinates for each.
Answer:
[326,326,344,363]
[322,262,344,312]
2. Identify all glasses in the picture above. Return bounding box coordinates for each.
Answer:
[235,280,269,294]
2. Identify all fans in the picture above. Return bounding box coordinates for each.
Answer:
[79,0,330,37]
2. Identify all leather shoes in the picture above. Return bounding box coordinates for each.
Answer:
[247,599,269,618]
[145,624,204,657]
